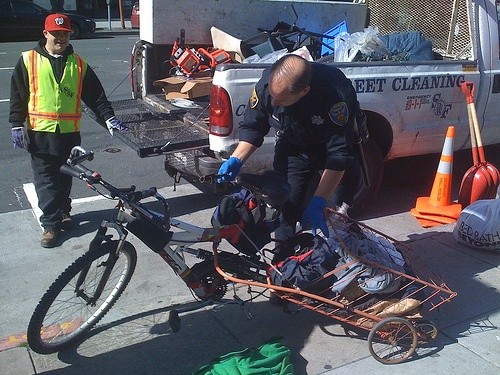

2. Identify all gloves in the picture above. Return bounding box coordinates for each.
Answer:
[11,126,26,149]
[216,157,243,184]
[106,114,132,136]
[301,195,329,239]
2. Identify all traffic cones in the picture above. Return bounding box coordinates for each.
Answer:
[409,124,463,230]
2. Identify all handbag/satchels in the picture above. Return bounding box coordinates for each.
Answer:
[210,185,280,255]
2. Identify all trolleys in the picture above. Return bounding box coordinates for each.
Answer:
[212,202,459,366]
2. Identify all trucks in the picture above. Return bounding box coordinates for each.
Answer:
[80,0,500,221]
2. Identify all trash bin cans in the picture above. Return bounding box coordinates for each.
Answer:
[131,2,140,29]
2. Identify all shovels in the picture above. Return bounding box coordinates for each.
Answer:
[458,81,500,211]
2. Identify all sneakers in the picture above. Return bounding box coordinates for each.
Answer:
[61,211,73,231]
[41,228,59,247]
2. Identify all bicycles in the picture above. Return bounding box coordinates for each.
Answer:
[22,143,339,356]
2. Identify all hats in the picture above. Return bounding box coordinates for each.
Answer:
[44,14,72,31]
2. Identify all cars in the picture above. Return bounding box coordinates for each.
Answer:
[129,2,140,29]
[0,0,97,44]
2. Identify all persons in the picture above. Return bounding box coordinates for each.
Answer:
[216,54,362,304]
[9,14,128,248]
